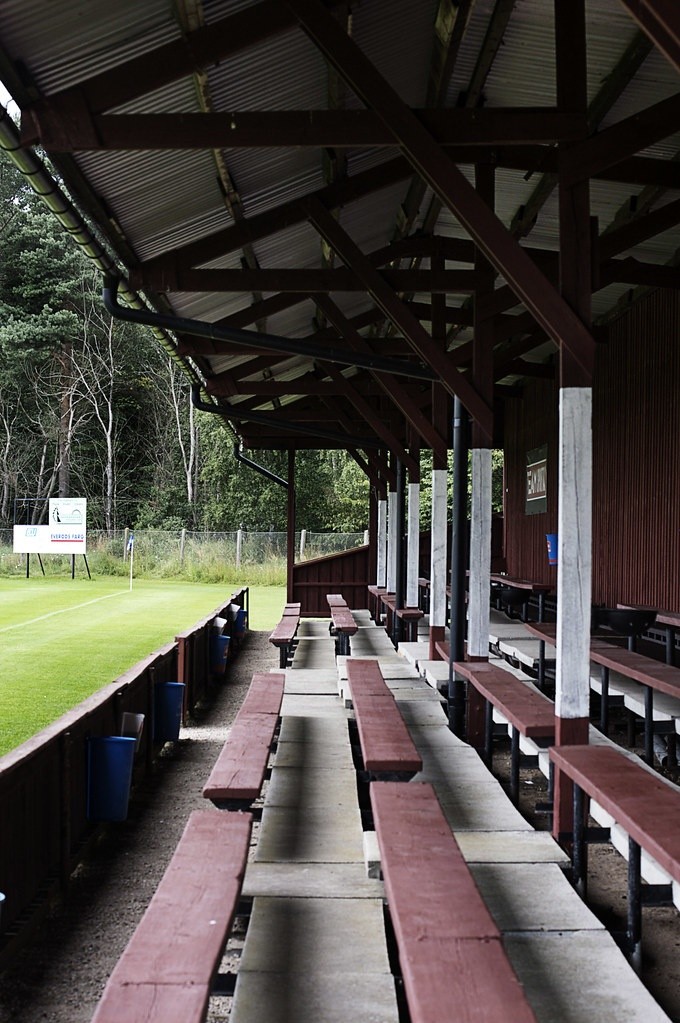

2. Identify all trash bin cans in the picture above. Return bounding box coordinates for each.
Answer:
[232,609,247,633]
[87,734,137,823]
[215,635,231,666]
[158,682,186,741]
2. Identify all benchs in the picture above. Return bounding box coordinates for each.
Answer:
[90,571,680,1023]
